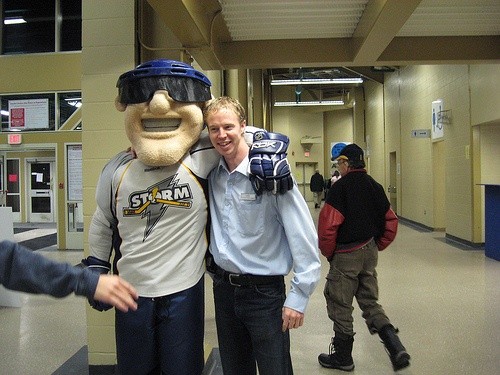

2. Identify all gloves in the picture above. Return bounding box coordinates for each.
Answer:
[82,256,115,312]
[249,131,293,195]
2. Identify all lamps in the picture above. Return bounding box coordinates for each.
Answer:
[3,10,32,25]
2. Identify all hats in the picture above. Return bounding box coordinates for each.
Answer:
[331,143,364,162]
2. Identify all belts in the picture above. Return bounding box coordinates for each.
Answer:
[214,268,283,286]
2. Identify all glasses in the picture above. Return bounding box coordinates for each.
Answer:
[337,159,346,167]
[118,75,213,104]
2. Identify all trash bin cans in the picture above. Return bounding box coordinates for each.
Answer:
[484,184,500,262]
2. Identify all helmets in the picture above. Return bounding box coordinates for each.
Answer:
[118,59,211,86]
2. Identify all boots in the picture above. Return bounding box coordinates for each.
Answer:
[379,324,411,372]
[318,336,355,371]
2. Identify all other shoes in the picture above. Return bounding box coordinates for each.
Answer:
[315,203,318,208]
[318,206,320,208]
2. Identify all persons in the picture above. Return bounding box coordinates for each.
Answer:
[310,169,325,208]
[0,239,138,313]
[204,96,321,375]
[317,144,411,372]
[331,170,341,183]
[82,59,294,375]
